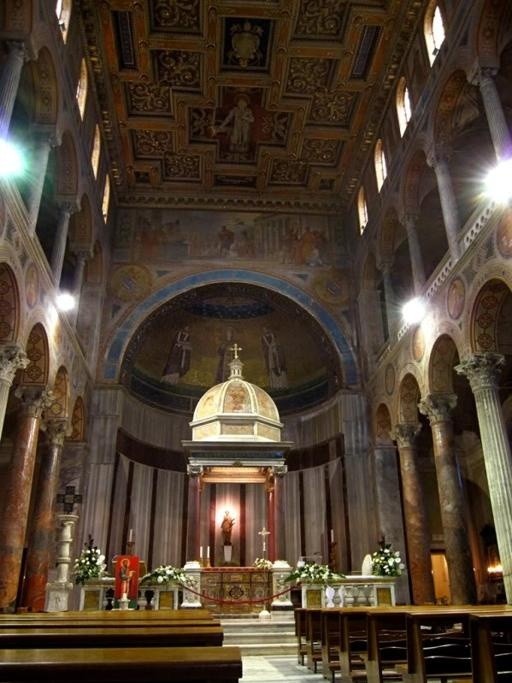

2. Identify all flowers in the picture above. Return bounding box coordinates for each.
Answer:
[284,560,346,591]
[370,543,406,576]
[138,564,198,588]
[72,548,111,586]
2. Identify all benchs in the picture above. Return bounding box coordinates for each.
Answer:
[294,605,512,683]
[0,609,242,682]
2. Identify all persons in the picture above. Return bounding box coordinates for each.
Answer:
[223,94,257,155]
[120,559,136,594]
[260,322,290,390]
[159,326,194,386]
[221,510,233,545]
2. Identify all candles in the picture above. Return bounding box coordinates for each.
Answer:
[129,529,132,542]
[263,541,266,551]
[331,528,334,543]
[200,545,210,558]
